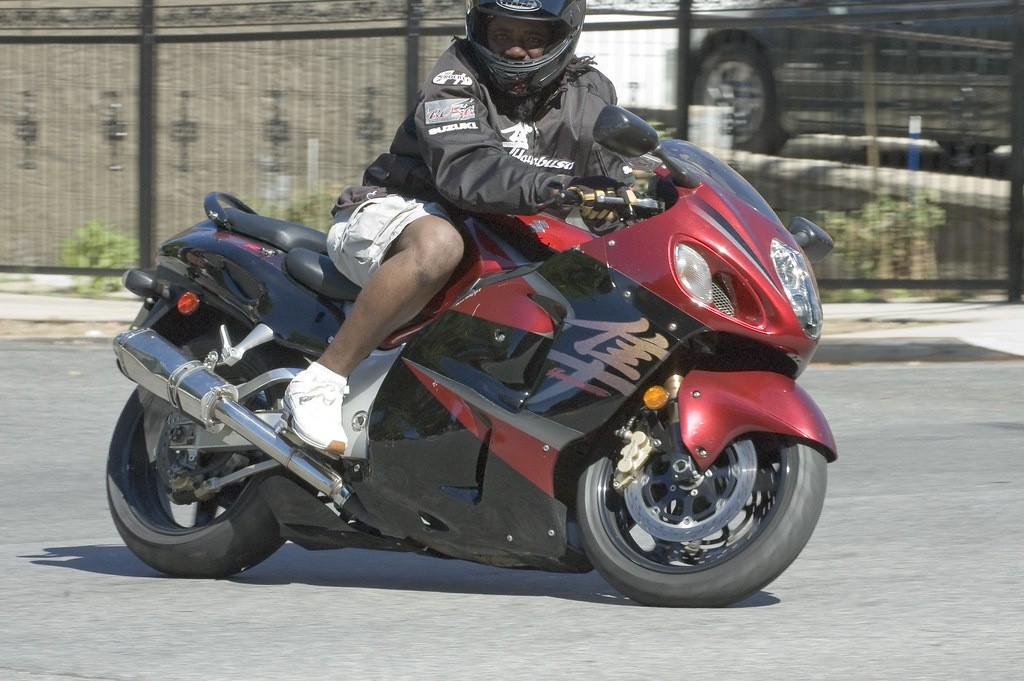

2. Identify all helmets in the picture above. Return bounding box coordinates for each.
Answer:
[465,0,586,97]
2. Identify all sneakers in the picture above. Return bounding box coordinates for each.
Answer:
[281,370,347,454]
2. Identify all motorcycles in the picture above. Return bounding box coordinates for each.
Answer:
[106,106,839,608]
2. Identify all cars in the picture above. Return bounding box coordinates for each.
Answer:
[685,0,1023,155]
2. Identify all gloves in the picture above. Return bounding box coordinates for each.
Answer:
[546,175,618,221]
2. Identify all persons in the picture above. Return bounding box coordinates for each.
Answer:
[282,0,640,454]
